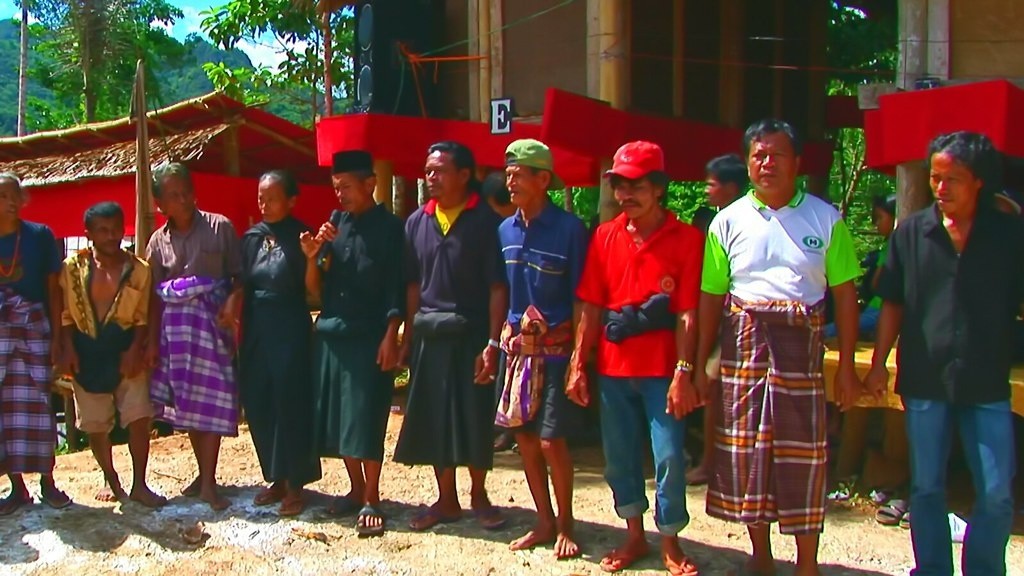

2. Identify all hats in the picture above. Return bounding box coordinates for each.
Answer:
[331,150,372,175]
[506,138,564,191]
[602,141,665,179]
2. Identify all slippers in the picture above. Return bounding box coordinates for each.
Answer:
[356,505,383,535]
[473,508,508,530]
[278,496,305,515]
[255,488,278,504]
[314,497,361,520]
[409,509,460,531]
[662,554,698,576]
[0,497,33,517]
[37,488,72,508]
[600,543,652,571]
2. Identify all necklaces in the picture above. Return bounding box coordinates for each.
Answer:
[0,220,20,276]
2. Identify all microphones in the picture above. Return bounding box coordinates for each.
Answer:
[316,210,341,268]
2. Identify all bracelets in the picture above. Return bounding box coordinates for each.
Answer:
[488,339,500,347]
[676,360,693,372]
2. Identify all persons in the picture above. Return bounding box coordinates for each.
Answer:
[141,164,241,511]
[498,138,589,557]
[825,192,897,436]
[396,141,510,529]
[223,170,324,516]
[688,153,750,485]
[567,141,707,576]
[58,202,168,507]
[316,150,406,535]
[865,129,1024,576]
[0,174,72,515]
[693,119,865,576]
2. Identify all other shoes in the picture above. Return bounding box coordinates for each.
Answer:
[948,512,967,541]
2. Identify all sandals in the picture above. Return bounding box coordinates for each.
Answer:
[874,498,906,524]
[900,507,910,529]
